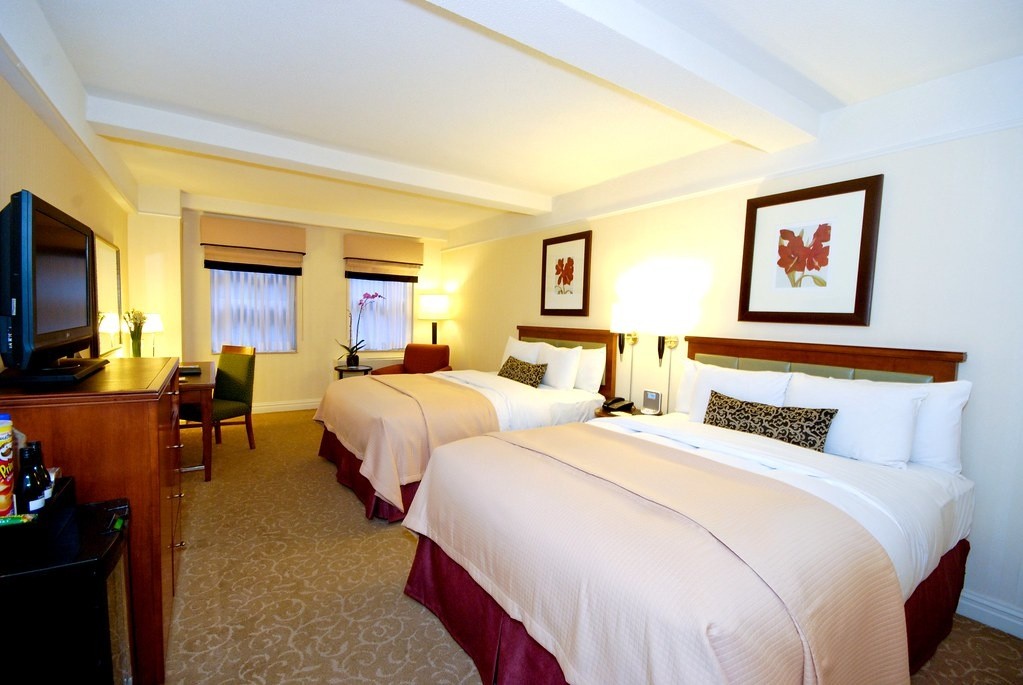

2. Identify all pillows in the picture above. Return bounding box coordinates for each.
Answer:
[499,336,547,375]
[573,344,609,393]
[494,356,548,389]
[786,373,924,462]
[537,345,582,390]
[702,390,838,453]
[675,358,790,413]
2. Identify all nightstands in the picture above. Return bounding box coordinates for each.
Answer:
[594,401,664,419]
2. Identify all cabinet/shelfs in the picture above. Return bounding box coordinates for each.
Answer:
[0,355,186,685]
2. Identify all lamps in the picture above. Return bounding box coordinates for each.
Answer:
[658,336,678,366]
[408,293,453,343]
[619,331,639,359]
[99,313,120,347]
[141,315,163,356]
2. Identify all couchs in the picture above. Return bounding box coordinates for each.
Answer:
[372,344,452,377]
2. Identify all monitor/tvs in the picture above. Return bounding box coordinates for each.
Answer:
[0,189,109,383]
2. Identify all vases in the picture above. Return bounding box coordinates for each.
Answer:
[347,352,359,366]
[131,328,145,359]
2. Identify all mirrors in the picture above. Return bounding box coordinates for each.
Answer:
[96,234,122,357]
[908,382,972,476]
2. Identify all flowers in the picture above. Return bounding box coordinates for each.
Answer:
[123,310,147,338]
[335,292,388,356]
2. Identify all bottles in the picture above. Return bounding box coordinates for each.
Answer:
[0,412,26,477]
[25,441,53,503]
[13,447,46,516]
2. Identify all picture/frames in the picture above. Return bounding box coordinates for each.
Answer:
[540,230,592,317]
[737,173,883,323]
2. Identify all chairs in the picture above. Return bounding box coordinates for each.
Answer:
[186,346,256,448]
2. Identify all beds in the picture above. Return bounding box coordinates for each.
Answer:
[315,325,620,525]
[402,335,976,685]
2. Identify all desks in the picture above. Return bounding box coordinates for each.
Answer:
[0,498,132,685]
[334,366,372,380]
[181,359,219,483]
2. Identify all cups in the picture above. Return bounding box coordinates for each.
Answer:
[0,420,14,523]
